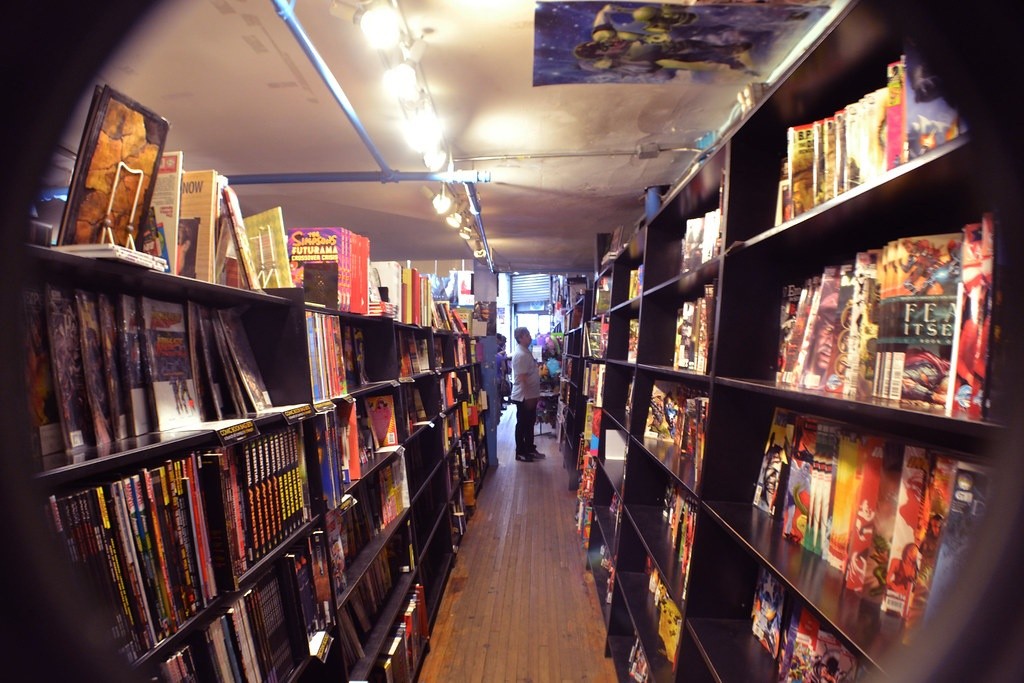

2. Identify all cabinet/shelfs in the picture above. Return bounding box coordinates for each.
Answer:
[23,1,999,683]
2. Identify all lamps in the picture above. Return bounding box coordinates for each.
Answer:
[371,39,426,94]
[419,179,452,216]
[330,4,397,47]
[458,214,476,239]
[445,200,469,226]
[474,240,487,257]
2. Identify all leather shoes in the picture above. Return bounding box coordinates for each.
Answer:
[529,451,545,459]
[516,455,533,463]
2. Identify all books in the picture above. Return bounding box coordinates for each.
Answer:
[147,529,334,683]
[774,212,994,423]
[327,460,402,598]
[334,533,429,683]
[432,297,487,535]
[19,283,273,458]
[772,44,988,230]
[305,311,366,404]
[672,282,716,375]
[671,169,725,272]
[557,275,709,683]
[750,406,995,659]
[48,427,314,664]
[137,149,294,293]
[287,223,431,327]
[744,567,874,683]
[396,328,427,435]
[316,394,398,511]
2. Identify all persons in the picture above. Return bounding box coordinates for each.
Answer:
[511,328,550,462]
[496,332,513,424]
